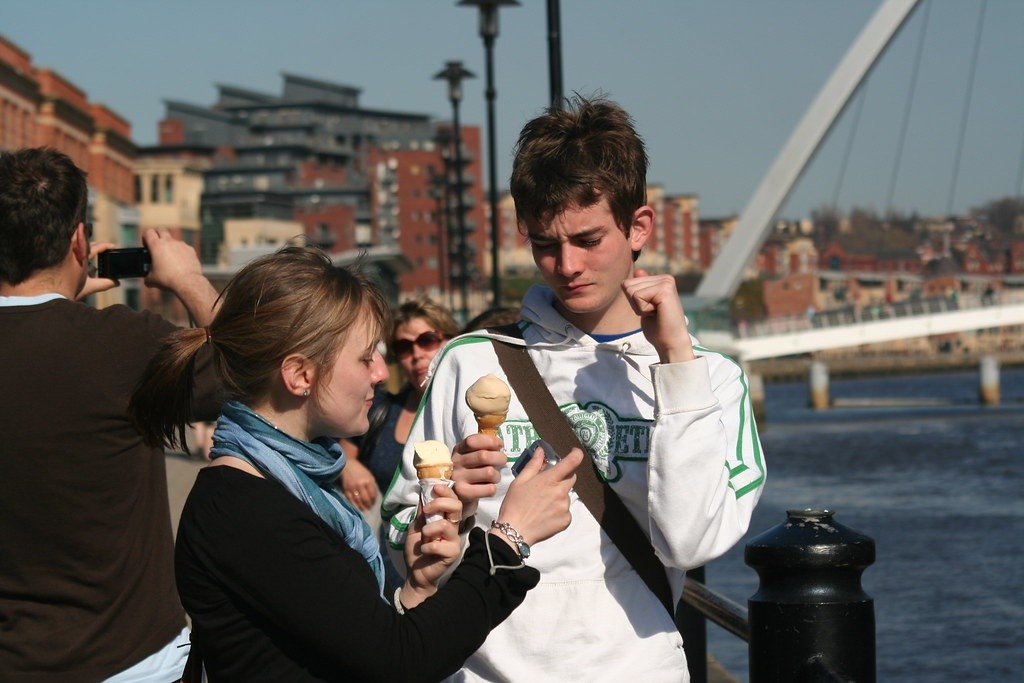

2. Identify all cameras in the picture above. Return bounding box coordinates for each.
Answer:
[97,247,153,279]
[511,438,564,479]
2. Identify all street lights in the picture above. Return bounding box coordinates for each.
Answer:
[432,61,477,326]
[452,0,521,310]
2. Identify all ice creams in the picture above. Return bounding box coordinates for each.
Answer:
[412,440,453,481]
[465,373,512,435]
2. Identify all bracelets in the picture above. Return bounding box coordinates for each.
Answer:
[483,530,526,576]
[491,520,531,558]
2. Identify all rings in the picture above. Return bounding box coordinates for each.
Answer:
[354,490,359,496]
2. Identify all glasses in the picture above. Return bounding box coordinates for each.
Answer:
[70,223,94,237]
[392,331,440,358]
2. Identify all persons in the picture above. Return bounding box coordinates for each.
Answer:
[128,246,584,683]
[378,103,769,683]
[193,421,213,461]
[336,293,459,533]
[0,145,226,683]
[465,307,520,334]
